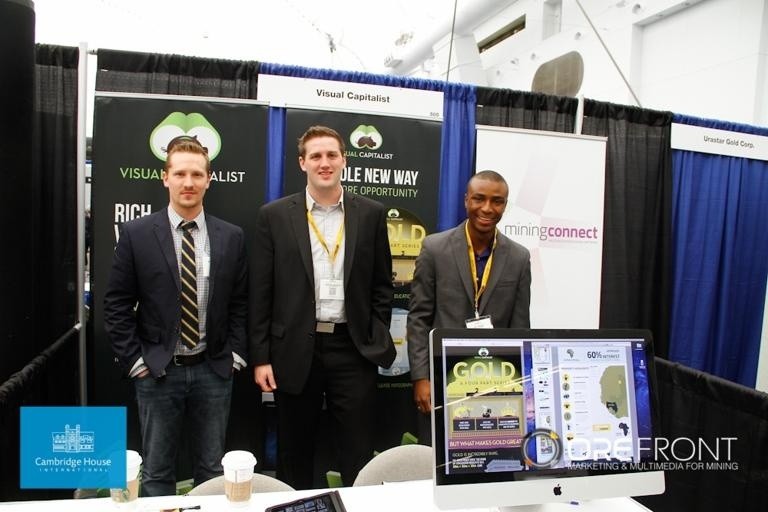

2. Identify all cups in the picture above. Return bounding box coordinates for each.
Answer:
[222,450,257,502]
[110,449,143,502]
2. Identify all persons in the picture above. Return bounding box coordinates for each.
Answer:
[249,123,397,488]
[100,140,251,495]
[398,168,536,449]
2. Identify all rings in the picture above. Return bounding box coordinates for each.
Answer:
[417,405,421,410]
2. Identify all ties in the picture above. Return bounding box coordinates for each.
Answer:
[178,221,201,352]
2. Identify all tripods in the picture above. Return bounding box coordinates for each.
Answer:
[429,328,665,512]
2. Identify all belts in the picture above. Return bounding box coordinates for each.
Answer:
[171,355,198,367]
[315,321,345,335]
